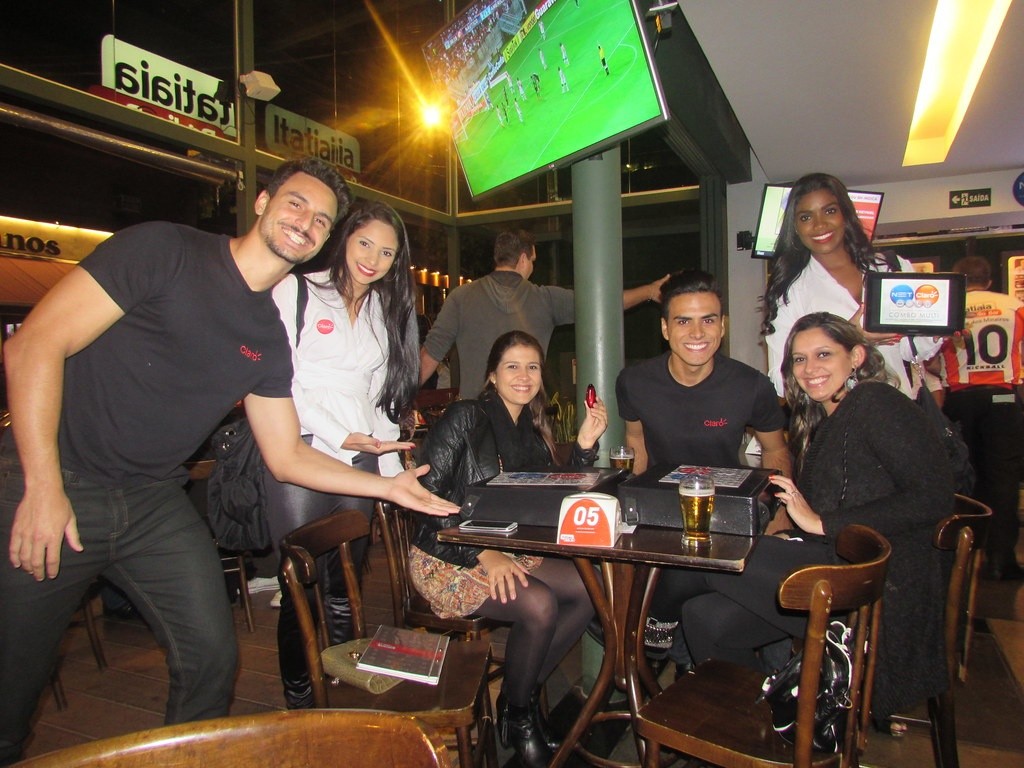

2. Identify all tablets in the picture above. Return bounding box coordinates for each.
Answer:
[865,271,966,335]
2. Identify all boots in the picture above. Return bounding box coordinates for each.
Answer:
[529,701,561,752]
[280,674,313,710]
[587,612,679,659]
[494,695,552,768]
[323,592,353,646]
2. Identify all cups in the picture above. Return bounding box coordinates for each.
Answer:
[679,476,715,548]
[609,446,635,474]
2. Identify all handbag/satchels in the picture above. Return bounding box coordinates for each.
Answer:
[204,418,273,552]
[752,615,867,754]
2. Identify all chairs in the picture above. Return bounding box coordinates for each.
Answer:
[634,526,892,768]
[867,494,993,768]
[377,499,511,768]
[278,508,498,767]
[0,709,451,768]
[183,459,255,632]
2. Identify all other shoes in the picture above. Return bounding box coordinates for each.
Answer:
[610,663,662,694]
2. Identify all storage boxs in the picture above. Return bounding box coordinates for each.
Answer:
[620,460,782,536]
[464,468,621,528]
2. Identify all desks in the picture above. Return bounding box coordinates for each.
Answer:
[437,508,758,768]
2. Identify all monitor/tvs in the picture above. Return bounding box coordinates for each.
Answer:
[751,183,885,260]
[421,0,673,200]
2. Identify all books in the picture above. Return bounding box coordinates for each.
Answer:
[357,625,449,684]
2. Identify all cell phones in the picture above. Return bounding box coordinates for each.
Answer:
[459,519,518,532]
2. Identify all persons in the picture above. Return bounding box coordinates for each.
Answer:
[405,331,606,767]
[614,267,794,681]
[757,174,977,506]
[924,257,1024,581]
[583,312,957,729]
[0,155,461,767]
[246,199,419,709]
[415,229,670,398]
